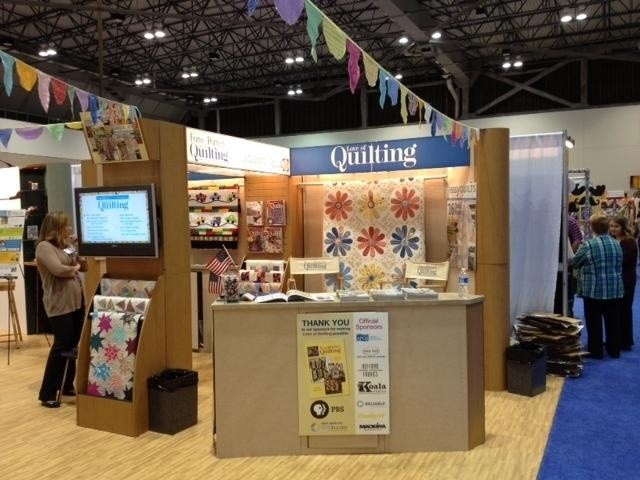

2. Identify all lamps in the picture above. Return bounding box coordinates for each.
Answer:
[562,134,578,151]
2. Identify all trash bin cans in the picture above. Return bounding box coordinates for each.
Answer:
[506,343,548,397]
[147,368,198,434]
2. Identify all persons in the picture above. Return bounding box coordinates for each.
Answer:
[609,215,637,350]
[568,213,623,359]
[555,214,583,318]
[33,211,86,407]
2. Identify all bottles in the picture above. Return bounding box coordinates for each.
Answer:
[457,267,471,298]
[223,270,239,304]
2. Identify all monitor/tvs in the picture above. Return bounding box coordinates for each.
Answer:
[73,183,158,259]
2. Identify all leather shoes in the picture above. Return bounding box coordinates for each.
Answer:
[41,400,60,408]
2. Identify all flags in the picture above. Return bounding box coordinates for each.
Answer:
[206,249,234,277]
[207,271,222,294]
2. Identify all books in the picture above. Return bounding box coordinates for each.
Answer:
[246,199,285,254]
[255,287,439,302]
